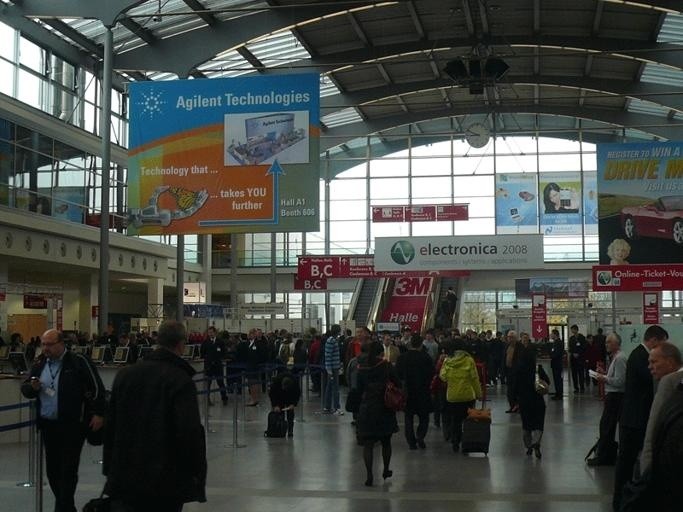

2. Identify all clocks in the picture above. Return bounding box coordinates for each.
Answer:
[465,121,489,148]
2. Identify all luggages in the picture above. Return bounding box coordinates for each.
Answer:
[462,400,491,456]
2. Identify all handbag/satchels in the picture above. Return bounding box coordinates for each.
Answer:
[466,409,490,421]
[80,391,112,445]
[346,391,361,412]
[534,373,548,394]
[594,439,617,465]
[81,492,118,512]
[385,383,408,411]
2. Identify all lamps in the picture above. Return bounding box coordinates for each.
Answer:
[440,41,513,96]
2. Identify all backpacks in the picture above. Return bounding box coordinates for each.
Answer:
[264,411,287,437]
[280,345,289,364]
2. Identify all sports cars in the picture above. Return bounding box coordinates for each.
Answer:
[620,195,683,242]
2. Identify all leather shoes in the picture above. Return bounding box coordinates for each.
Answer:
[208,402,214,406]
[222,400,228,406]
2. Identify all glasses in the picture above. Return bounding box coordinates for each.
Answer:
[42,342,60,346]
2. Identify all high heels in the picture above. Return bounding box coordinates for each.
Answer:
[365,478,373,486]
[247,402,258,406]
[382,470,393,480]
[526,448,533,456]
[535,446,541,458]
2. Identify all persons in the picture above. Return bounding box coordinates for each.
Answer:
[606,238,632,266]
[188,323,355,439]
[541,182,579,215]
[0,325,159,373]
[100,318,208,510]
[645,342,683,380]
[345,326,565,486]
[446,287,457,322]
[18,327,105,510]
[590,329,627,468]
[636,366,683,511]
[568,324,606,394]
[611,324,670,511]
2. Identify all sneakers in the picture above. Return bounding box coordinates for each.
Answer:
[574,389,579,394]
[551,395,563,400]
[580,389,585,394]
[434,423,440,429]
[453,440,459,451]
[588,457,604,467]
[334,409,345,416]
[607,456,615,465]
[323,408,334,415]
[417,438,425,448]
[505,408,513,412]
[493,383,497,387]
[446,431,453,439]
[409,444,417,449]
[515,406,520,412]
[486,384,491,388]
